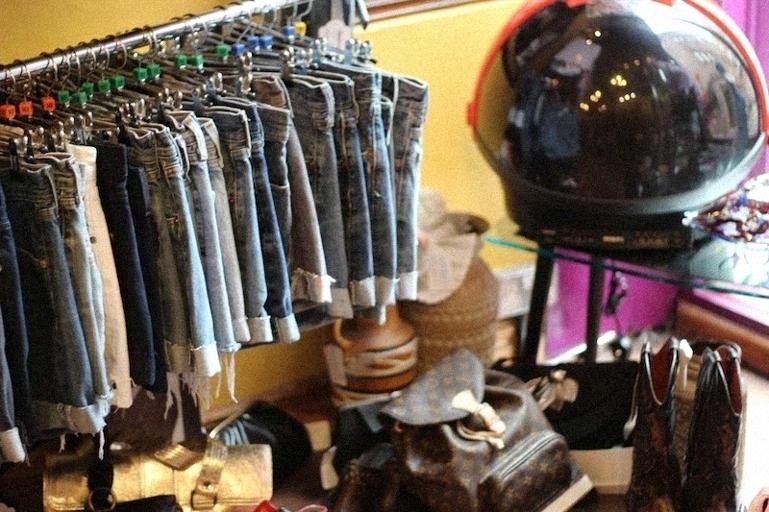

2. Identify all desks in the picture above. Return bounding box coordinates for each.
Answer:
[482,174,769,365]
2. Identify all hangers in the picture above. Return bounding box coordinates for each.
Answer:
[0,0,379,161]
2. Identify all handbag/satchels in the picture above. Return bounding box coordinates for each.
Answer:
[623,336,682,512]
[326,352,598,512]
[682,340,747,512]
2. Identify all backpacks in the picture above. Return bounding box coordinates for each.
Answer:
[377,345,595,512]
